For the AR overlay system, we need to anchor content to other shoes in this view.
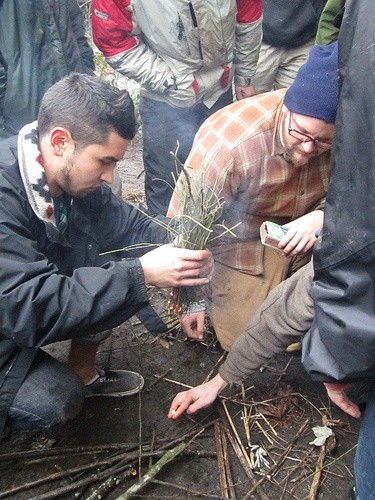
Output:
[285,340,301,352]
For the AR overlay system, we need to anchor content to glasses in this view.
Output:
[287,110,333,150]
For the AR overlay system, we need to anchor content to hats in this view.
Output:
[282,40,339,123]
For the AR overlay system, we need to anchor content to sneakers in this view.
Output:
[82,366,145,396]
[7,428,58,450]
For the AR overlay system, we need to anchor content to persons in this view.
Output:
[297,0,375,500]
[0,72,215,445]
[166,258,317,419]
[250,0,328,94]
[0,0,123,200]
[89,0,264,219]
[165,40,342,352]
